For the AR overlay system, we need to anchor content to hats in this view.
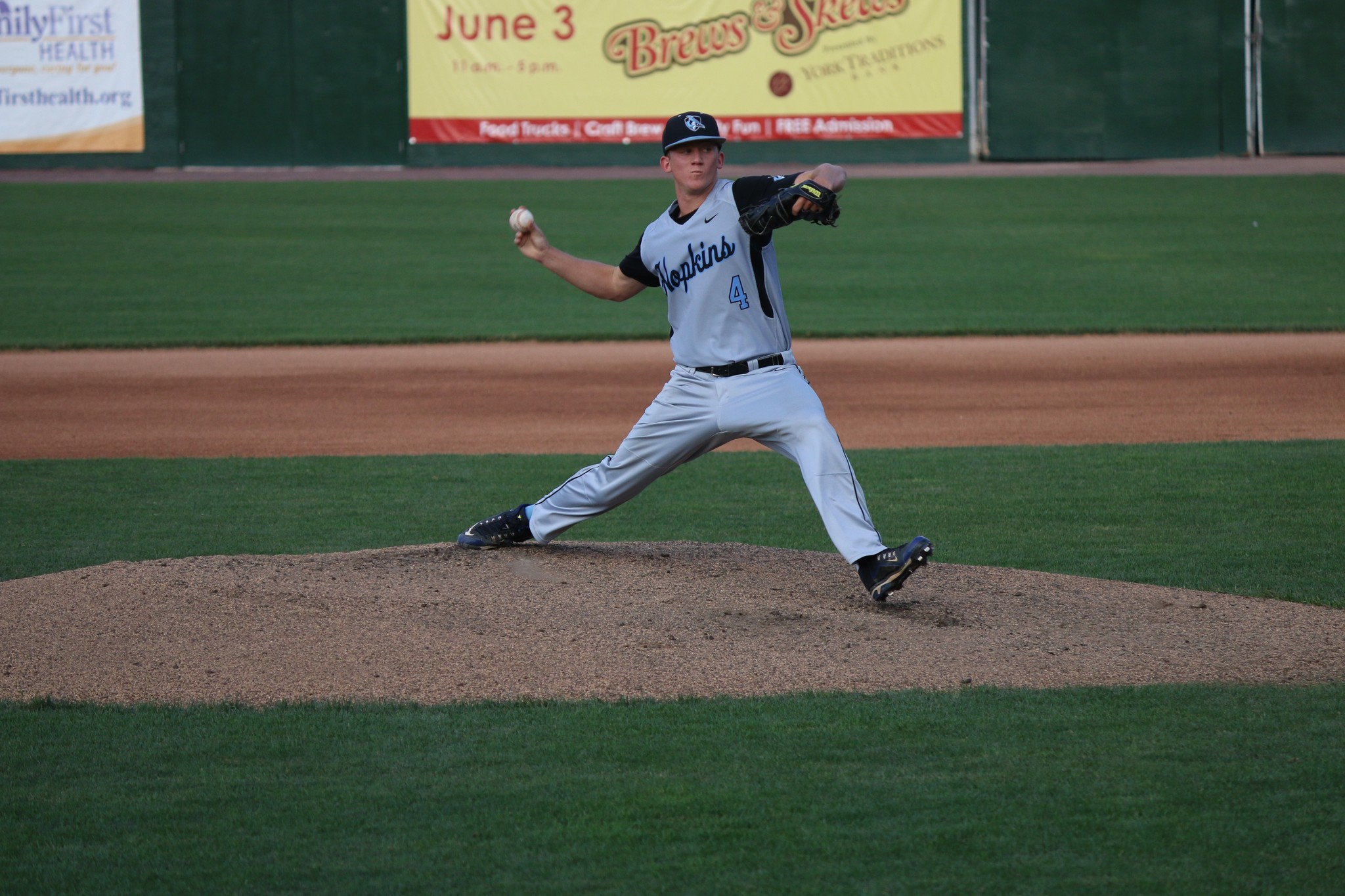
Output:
[662,111,727,155]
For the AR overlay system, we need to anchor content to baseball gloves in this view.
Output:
[738,179,840,244]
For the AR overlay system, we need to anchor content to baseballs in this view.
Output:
[510,207,533,234]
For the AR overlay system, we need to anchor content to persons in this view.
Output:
[456,109,935,602]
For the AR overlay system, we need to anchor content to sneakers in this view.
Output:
[855,536,935,602]
[457,504,534,550]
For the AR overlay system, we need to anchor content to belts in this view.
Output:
[685,354,784,377]
